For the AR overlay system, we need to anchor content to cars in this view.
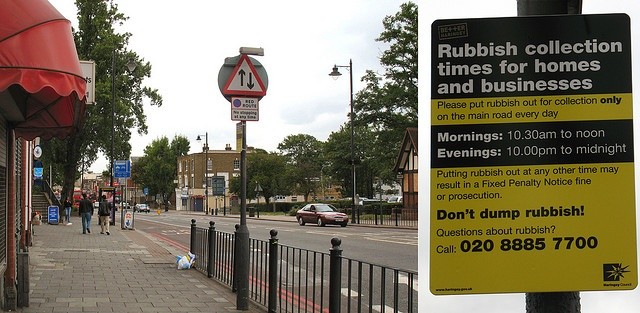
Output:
[134,203,150,213]
[297,204,348,227]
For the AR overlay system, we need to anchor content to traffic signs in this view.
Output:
[231,97,259,121]
[114,160,130,178]
[34,168,43,177]
[48,205,59,223]
[144,188,148,195]
[218,55,268,102]
[223,54,266,96]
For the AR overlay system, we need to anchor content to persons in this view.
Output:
[97,195,112,236]
[78,193,94,234]
[64,197,72,223]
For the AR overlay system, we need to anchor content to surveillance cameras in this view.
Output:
[239,47,264,56]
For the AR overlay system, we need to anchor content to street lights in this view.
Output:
[196,132,208,214]
[329,59,356,223]
